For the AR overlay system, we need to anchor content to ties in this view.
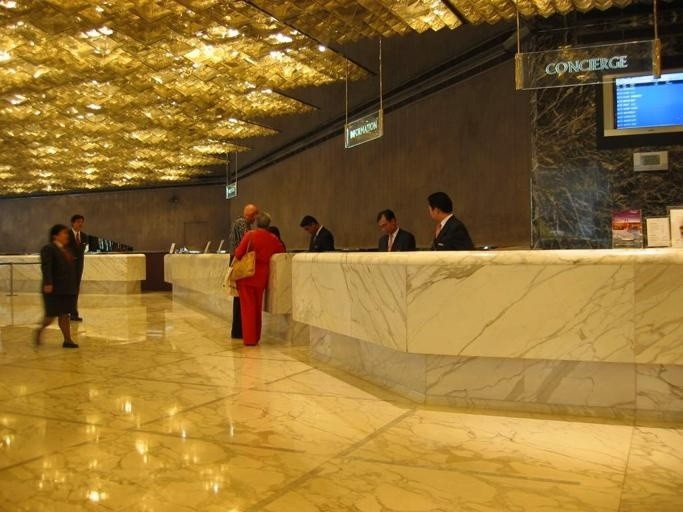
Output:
[388,234,393,251]
[76,232,80,247]
[435,223,441,238]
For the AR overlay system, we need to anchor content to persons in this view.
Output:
[64,214,87,321]
[426,192,475,250]
[228,204,286,346]
[300,215,334,251]
[377,210,415,251]
[31,224,79,348]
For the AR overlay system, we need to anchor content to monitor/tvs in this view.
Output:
[601,67,683,137]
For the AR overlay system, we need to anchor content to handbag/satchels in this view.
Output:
[229,250,257,281]
[222,268,240,297]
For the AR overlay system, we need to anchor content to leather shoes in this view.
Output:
[62,341,79,348]
[70,315,82,322]
